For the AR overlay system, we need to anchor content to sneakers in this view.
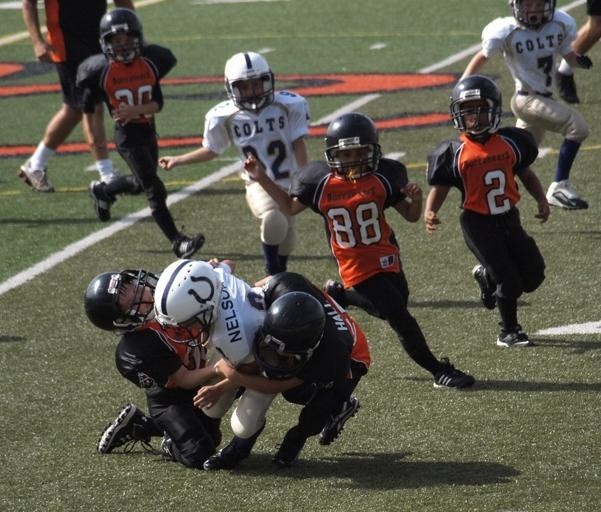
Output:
[171,232,205,260]
[545,178,589,210]
[554,64,581,104]
[15,163,55,193]
[318,395,360,446]
[324,278,346,311]
[89,179,117,223]
[472,263,498,310]
[158,411,222,460]
[275,425,307,466]
[496,327,534,348]
[96,403,150,455]
[432,362,476,390]
[203,419,267,472]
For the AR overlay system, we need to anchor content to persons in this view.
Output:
[551,0,601,103]
[153,255,280,472]
[242,112,477,391]
[76,7,204,261]
[208,273,372,469]
[157,51,310,278]
[85,268,220,470]
[457,0,590,210]
[17,0,137,194]
[423,73,550,349]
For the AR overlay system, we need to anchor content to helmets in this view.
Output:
[252,291,327,381]
[223,50,276,112]
[323,113,383,184]
[84,268,160,334]
[97,7,145,65]
[508,0,558,31]
[449,76,502,142]
[152,258,222,351]
